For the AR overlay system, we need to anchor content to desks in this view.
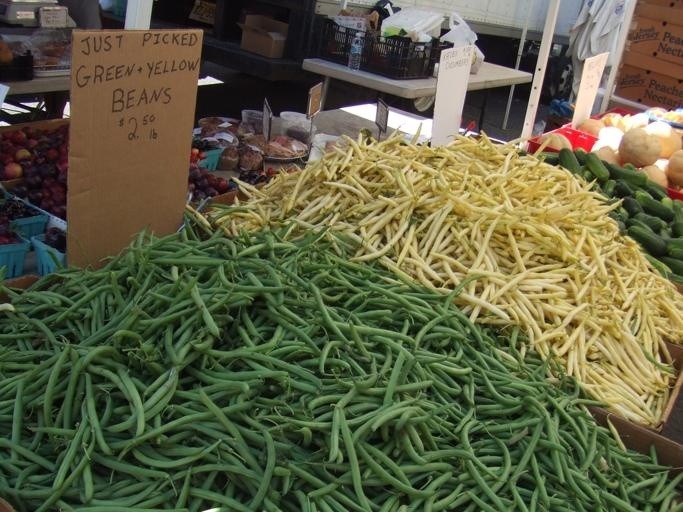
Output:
[306,58,533,135]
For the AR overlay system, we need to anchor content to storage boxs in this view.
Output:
[586,336,683,469]
[612,0,683,118]
[235,12,290,60]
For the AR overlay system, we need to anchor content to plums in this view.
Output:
[0,125,69,220]
[187,147,236,204]
[239,166,276,187]
[191,139,218,152]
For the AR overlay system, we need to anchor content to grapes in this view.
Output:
[0,198,42,226]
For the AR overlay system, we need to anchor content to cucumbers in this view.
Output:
[539,147,683,284]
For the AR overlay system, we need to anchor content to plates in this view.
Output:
[246,137,310,160]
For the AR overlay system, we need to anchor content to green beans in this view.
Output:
[0,196,682,512]
[185,119,682,432]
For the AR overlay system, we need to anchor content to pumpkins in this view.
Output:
[537,133,573,152]
[576,106,683,136]
[596,121,683,188]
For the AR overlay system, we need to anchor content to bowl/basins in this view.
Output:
[280,112,308,121]
[242,110,267,133]
[284,122,317,141]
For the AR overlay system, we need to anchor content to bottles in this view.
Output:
[346,32,363,72]
